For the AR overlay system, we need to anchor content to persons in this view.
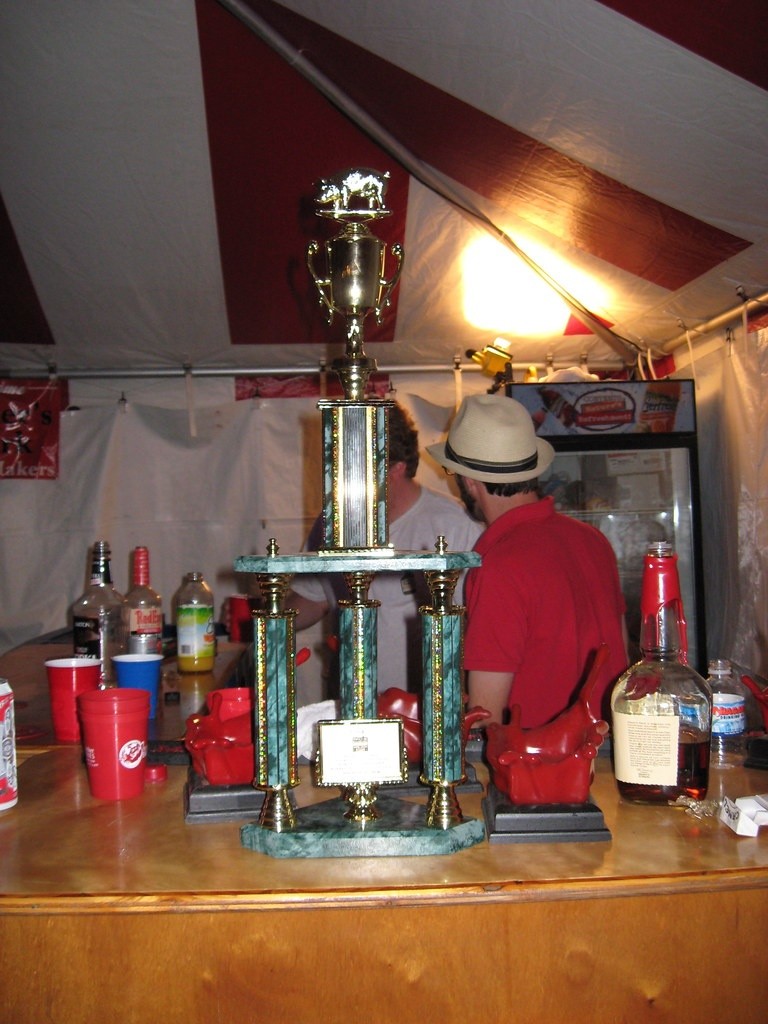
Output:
[463,396,630,729]
[224,395,484,693]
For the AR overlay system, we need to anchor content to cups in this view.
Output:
[45,658,102,742]
[111,654,165,720]
[207,687,255,718]
[79,688,151,800]
[230,597,261,642]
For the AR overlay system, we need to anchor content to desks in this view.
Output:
[0,619,767,1024]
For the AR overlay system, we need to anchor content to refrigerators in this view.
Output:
[505,379,706,686]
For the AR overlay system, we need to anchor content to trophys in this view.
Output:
[307,167,405,401]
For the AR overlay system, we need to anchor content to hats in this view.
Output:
[424,395,556,483]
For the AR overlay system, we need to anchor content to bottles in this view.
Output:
[531,407,547,432]
[611,540,713,806]
[546,354,554,375]
[537,386,580,429]
[636,382,681,433]
[120,546,162,654]
[708,659,747,768]
[176,572,216,674]
[72,542,130,687]
[580,351,588,374]
